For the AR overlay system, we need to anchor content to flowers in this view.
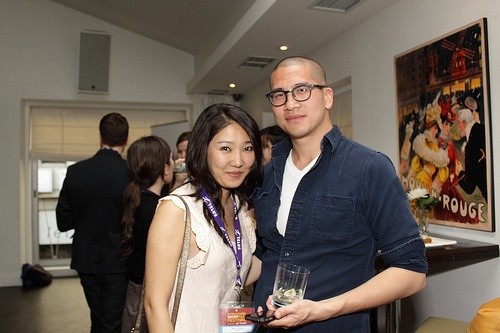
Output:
[407,187,429,211]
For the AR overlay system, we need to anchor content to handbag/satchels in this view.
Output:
[21,264,52,289]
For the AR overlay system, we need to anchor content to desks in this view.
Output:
[371,233,500,333]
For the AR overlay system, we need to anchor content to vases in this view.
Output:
[417,210,430,240]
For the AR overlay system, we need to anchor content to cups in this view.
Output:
[272,262,311,309]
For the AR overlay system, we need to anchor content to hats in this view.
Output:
[465,97,477,110]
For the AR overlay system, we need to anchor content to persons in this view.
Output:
[56,112,134,333]
[230,57,429,333]
[160,125,293,198]
[143,103,257,333]
[121,135,174,285]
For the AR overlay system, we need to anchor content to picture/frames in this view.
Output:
[393,17,496,233]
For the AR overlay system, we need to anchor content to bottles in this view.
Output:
[171,161,188,174]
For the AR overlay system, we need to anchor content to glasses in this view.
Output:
[266,85,329,107]
